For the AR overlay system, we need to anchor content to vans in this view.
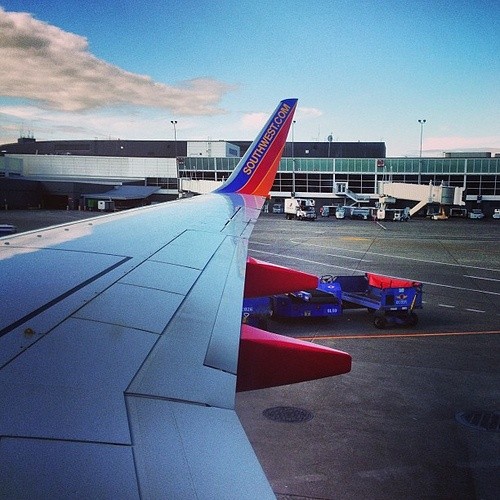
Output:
[273,203,282,214]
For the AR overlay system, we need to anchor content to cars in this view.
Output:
[321,203,499,224]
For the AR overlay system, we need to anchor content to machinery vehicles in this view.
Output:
[240,250,425,331]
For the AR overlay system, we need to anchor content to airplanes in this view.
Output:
[0,96,298,500]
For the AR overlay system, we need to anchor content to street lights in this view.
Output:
[170,119,178,177]
[418,119,426,183]
[289,120,296,194]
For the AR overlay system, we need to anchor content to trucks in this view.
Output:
[284,197,317,222]
